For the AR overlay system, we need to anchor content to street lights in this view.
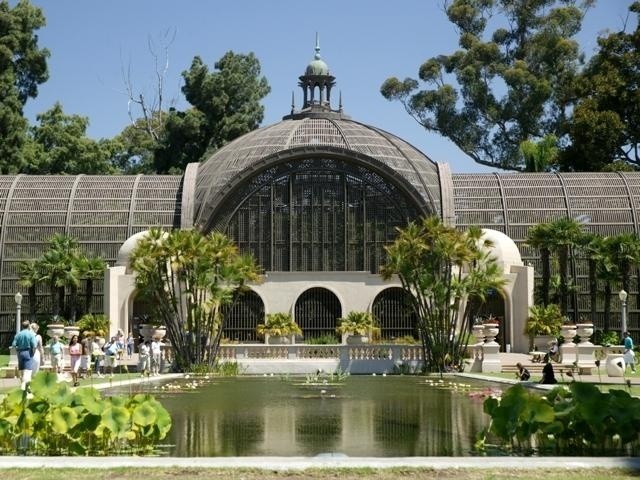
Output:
[15,292,22,332]
[619,290,627,338]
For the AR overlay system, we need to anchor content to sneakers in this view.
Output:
[69,368,162,387]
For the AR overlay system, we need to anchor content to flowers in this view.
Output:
[471,315,499,325]
[523,304,561,353]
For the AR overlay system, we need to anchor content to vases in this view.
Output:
[470,323,499,343]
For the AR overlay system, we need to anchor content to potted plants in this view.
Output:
[333,311,382,344]
[48,312,110,353]
[558,318,594,343]
[138,319,167,342]
[254,311,304,344]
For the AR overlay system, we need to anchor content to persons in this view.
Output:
[12,319,38,399]
[162,334,171,363]
[30,323,44,382]
[138,340,151,377]
[515,362,530,381]
[541,341,558,363]
[151,336,162,377]
[623,332,636,373]
[48,329,135,387]
